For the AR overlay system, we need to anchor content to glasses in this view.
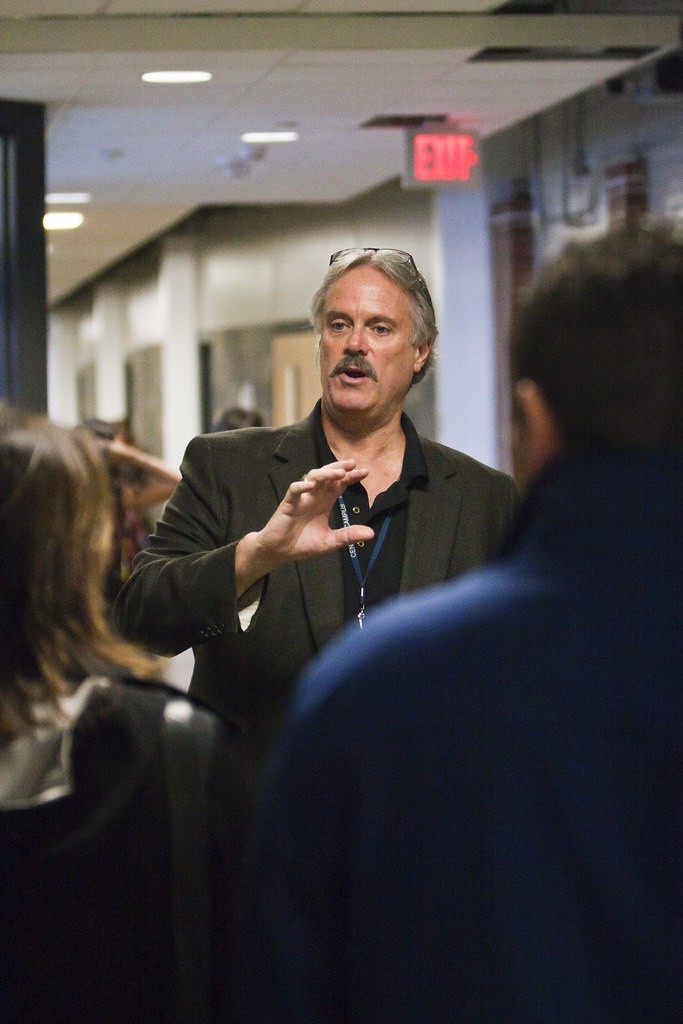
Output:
[328,248,421,280]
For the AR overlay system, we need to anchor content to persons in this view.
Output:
[228,210,682,1024]
[0,402,256,1024]
[108,247,524,776]
